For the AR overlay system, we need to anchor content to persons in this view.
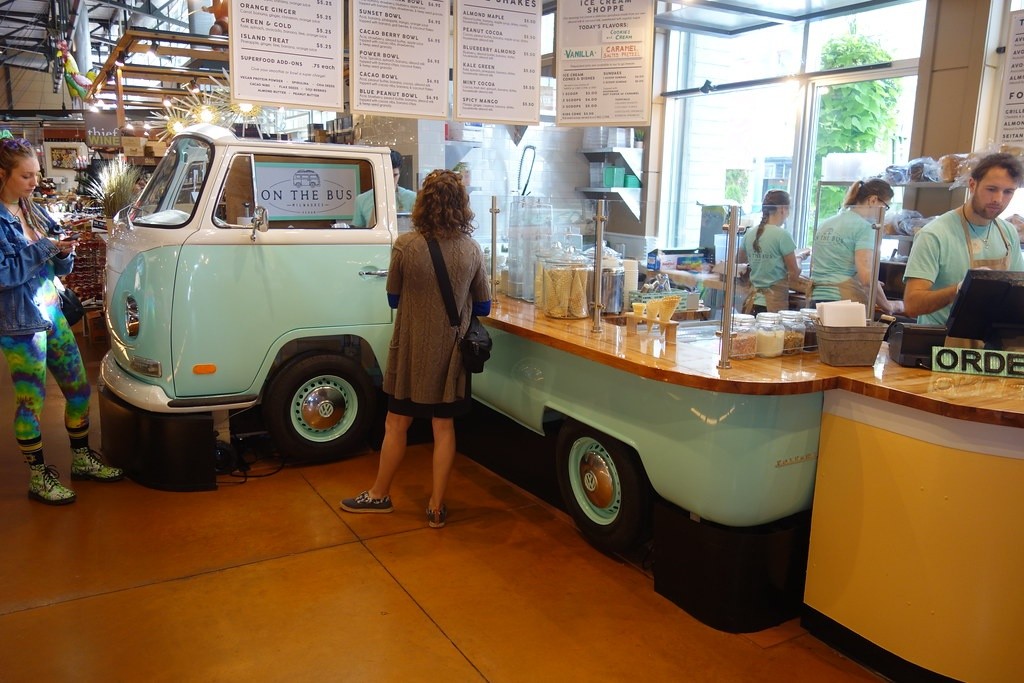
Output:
[0,130,126,506]
[348,150,417,229]
[806,179,905,324]
[903,153,1024,326]
[735,189,810,316]
[339,169,492,528]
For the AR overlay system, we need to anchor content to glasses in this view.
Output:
[868,194,890,212]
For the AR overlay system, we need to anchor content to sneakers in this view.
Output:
[70,445,124,483]
[27,465,77,505]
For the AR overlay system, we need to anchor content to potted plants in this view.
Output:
[79,151,148,239]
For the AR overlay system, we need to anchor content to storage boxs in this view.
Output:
[713,235,740,266]
[647,246,705,277]
[806,313,897,366]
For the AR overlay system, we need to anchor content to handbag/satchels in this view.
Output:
[459,315,493,374]
[58,288,85,326]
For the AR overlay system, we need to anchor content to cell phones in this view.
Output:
[63,233,81,242]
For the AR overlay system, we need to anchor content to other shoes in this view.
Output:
[426,504,447,527]
[340,491,394,513]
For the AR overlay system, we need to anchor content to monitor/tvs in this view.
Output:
[946,268,1024,353]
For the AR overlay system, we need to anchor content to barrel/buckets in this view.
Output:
[714,235,740,264]
[808,313,895,367]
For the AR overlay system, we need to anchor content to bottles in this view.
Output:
[728,308,818,360]
[534,241,624,319]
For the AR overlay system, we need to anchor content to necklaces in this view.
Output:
[0,194,24,223]
[963,204,992,249]
[0,198,19,204]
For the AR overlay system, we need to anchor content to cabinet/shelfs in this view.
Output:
[814,180,971,303]
